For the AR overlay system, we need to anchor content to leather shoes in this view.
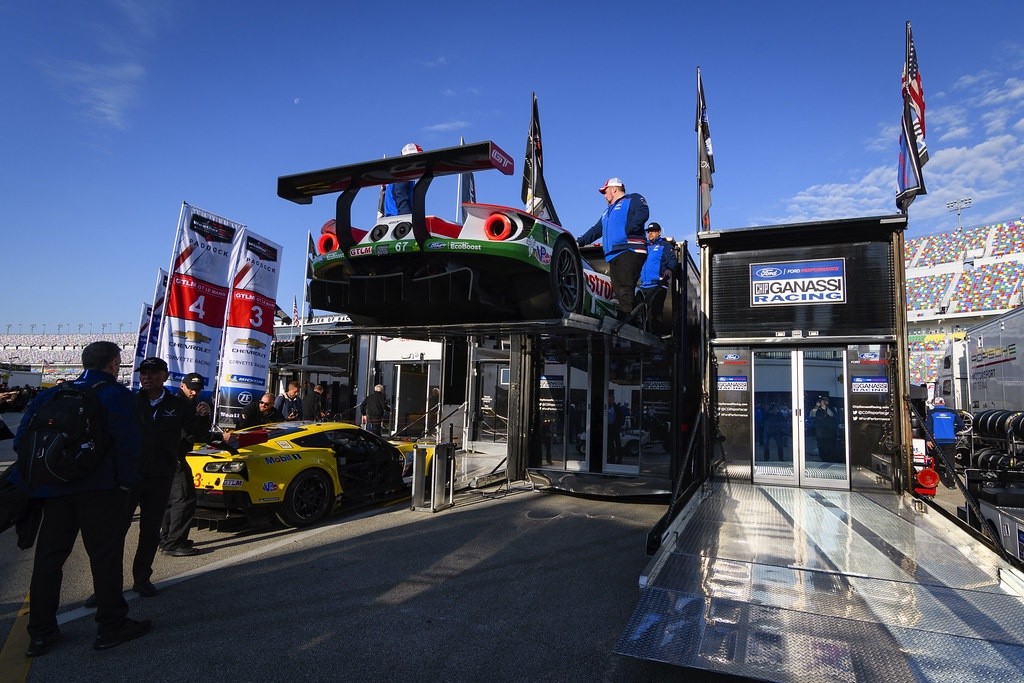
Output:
[159,540,193,551]
[94,619,153,648]
[133,580,158,596]
[163,545,199,555]
[85,592,97,608]
[26,625,60,654]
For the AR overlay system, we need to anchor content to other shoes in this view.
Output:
[949,485,958,490]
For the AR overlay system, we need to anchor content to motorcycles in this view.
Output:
[575,416,655,458]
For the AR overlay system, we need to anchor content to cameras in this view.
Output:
[818,395,822,402]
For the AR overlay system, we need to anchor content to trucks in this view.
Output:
[931,306,1024,443]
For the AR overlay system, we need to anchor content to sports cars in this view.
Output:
[184,420,459,535]
[276,141,624,321]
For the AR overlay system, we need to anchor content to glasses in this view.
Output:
[259,400,271,405]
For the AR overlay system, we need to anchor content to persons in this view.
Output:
[302,385,326,420]
[359,384,391,436]
[11,340,151,656]
[925,397,964,491]
[382,142,424,215]
[569,390,630,464]
[754,402,791,461]
[85,357,211,608]
[55,378,65,385]
[575,177,650,324]
[636,223,677,334]
[275,381,304,420]
[158,372,239,556]
[811,396,838,461]
[237,392,284,431]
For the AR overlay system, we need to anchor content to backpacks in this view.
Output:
[20,380,115,485]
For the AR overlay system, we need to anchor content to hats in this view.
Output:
[182,371,204,389]
[645,223,661,230]
[934,397,945,405]
[134,356,167,372]
[599,177,624,194]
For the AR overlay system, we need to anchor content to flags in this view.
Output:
[895,25,930,214]
[304,234,319,321]
[461,171,476,223]
[293,300,299,328]
[694,76,715,240]
[43,360,49,368]
[521,97,559,226]
